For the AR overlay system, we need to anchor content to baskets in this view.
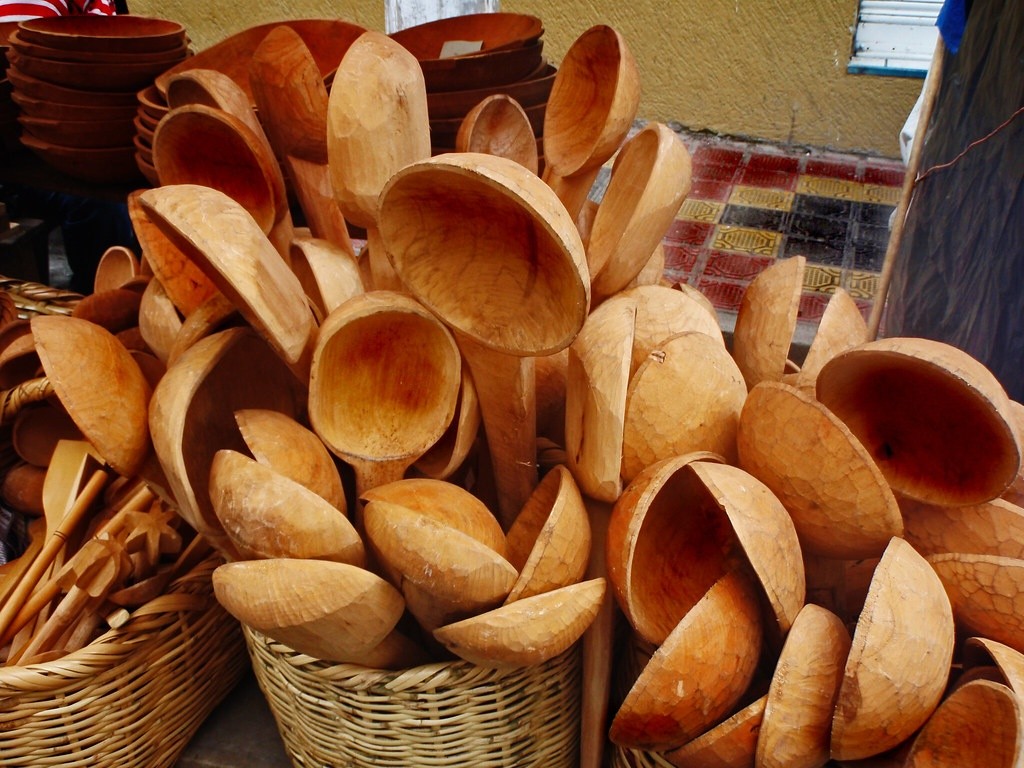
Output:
[239,618,582,768]
[607,622,671,767]
[0,549,246,768]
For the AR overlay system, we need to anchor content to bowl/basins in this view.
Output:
[0,13,563,242]
